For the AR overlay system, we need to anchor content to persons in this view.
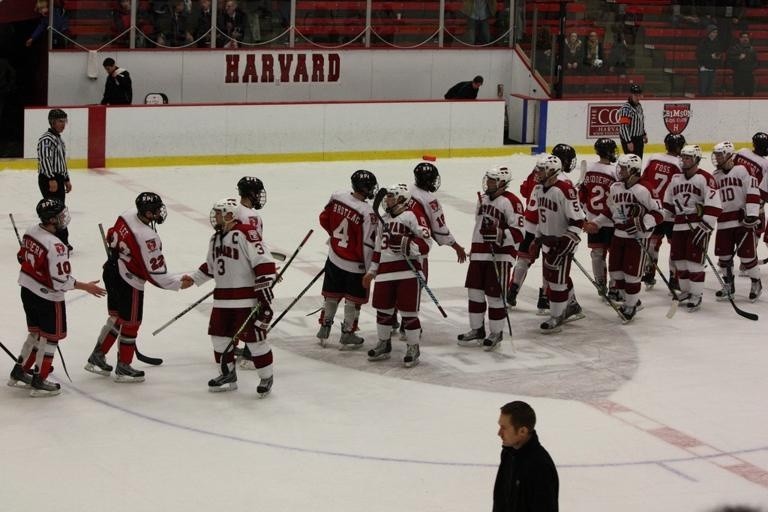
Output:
[179,176,284,398]
[84,193,189,382]
[463,1,758,101]
[116,0,289,52]
[8,199,108,397]
[99,57,133,106]
[37,109,74,252]
[445,76,483,100]
[458,84,768,352]
[493,401,558,512]
[26,2,73,50]
[317,162,465,366]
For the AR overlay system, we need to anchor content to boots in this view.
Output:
[10,355,33,384]
[538,288,583,329]
[87,342,113,371]
[257,375,273,392]
[458,282,520,350]
[749,277,762,299]
[32,365,61,391]
[317,320,422,364]
[115,352,145,377]
[208,358,237,386]
[716,276,735,297]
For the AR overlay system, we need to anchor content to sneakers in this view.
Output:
[669,271,702,308]
[598,274,656,320]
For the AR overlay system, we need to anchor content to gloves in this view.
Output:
[743,218,762,232]
[252,279,274,331]
[388,235,410,256]
[479,218,499,243]
[624,203,647,236]
[690,222,713,249]
[558,231,581,256]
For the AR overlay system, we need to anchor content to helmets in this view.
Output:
[534,144,577,185]
[482,165,511,193]
[237,176,267,209]
[48,109,68,127]
[594,138,645,190]
[665,132,768,172]
[36,198,71,231]
[209,199,239,235]
[351,162,442,214]
[135,192,167,224]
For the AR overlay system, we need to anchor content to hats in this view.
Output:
[631,84,642,93]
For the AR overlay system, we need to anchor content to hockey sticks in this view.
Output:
[372,188,448,320]
[637,239,684,319]
[219,230,312,374]
[98,223,163,366]
[675,199,757,321]
[9,214,74,384]
[152,251,287,339]
[477,190,515,349]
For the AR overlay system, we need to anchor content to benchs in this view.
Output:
[514,1,767,100]
[62,2,509,47]
